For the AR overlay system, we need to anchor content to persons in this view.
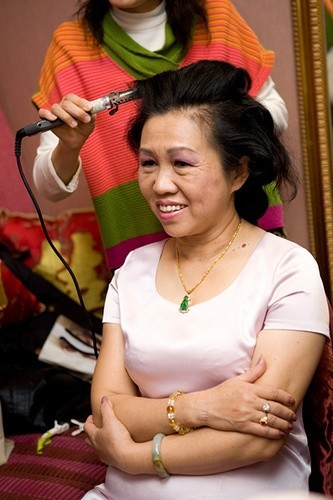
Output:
[76,56,333,500]
[27,0,297,293]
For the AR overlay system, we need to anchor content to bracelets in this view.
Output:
[151,431,172,480]
[164,390,194,435]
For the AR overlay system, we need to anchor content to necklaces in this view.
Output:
[174,217,244,314]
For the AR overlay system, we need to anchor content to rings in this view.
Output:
[259,412,270,426]
[262,400,271,413]
